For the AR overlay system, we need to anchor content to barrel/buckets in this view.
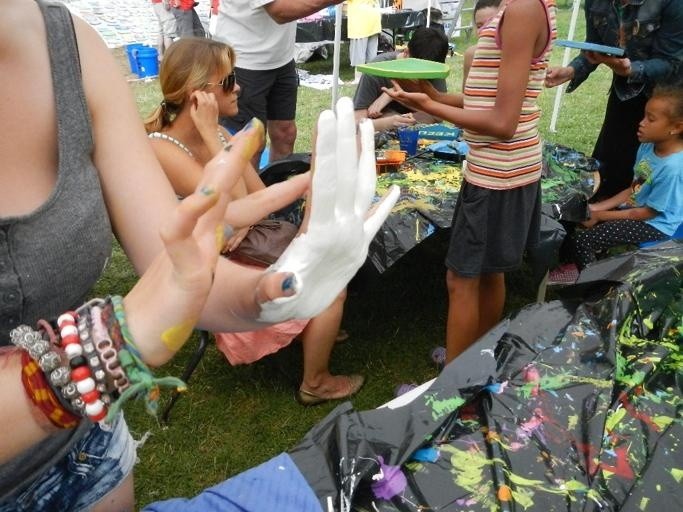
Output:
[135,47,158,79]
[127,43,142,72]
[257,146,270,170]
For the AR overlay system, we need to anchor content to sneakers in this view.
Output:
[429,347,447,364]
[394,383,418,397]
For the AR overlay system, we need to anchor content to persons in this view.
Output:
[145,2,365,407]
[382,0,556,399]
[1,0,401,512]
[347,1,450,129]
[542,1,683,289]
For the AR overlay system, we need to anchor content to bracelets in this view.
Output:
[12,294,157,436]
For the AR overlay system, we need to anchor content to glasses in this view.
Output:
[205,70,237,95]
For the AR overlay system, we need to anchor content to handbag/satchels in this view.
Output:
[229,218,299,267]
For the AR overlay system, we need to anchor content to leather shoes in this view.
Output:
[296,374,364,408]
